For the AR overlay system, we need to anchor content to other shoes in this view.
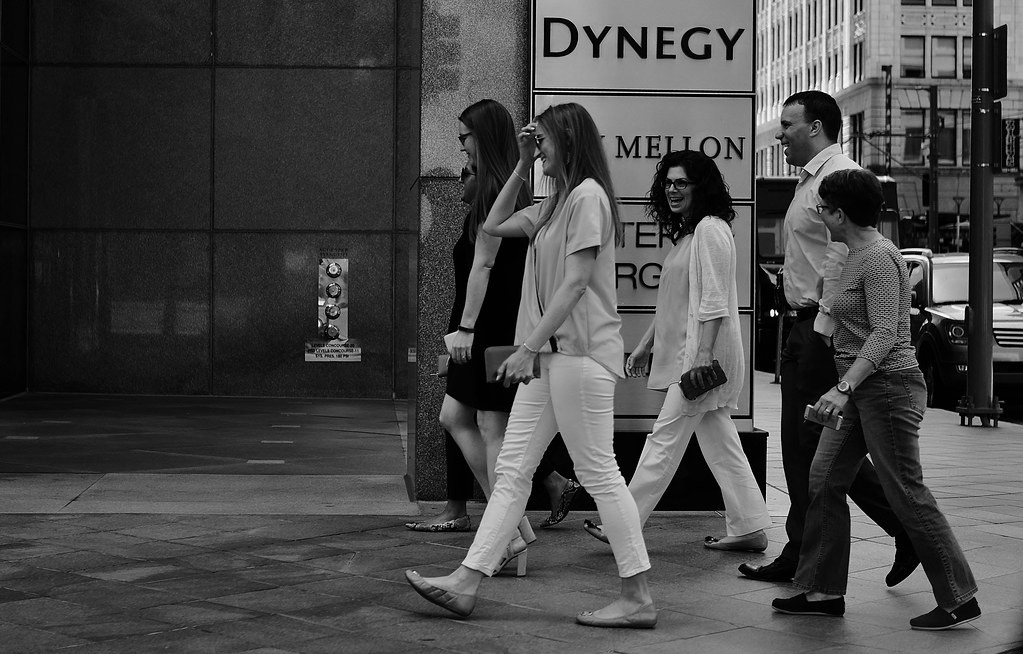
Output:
[405,516,471,533]
[540,478,586,527]
[583,520,611,545]
[885,529,921,586]
[403,571,475,617]
[739,564,794,581]
[704,531,768,551]
[577,603,658,628]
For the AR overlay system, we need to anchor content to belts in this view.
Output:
[789,304,821,321]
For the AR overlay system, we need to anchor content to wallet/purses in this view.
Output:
[438,355,451,376]
[680,361,727,400]
[483,346,541,383]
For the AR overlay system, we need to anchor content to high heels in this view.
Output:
[491,534,527,577]
[518,518,536,545]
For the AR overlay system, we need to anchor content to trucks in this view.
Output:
[754,171,905,371]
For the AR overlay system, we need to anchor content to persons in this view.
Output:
[584,150,773,552]
[412,99,578,576]
[771,168,982,630]
[740,89,919,587]
[406,104,658,629]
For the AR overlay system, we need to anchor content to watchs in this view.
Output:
[835,380,853,396]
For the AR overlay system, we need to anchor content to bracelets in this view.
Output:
[513,171,526,183]
[456,326,477,335]
[523,342,540,354]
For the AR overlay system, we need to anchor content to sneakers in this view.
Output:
[769,592,845,616]
[910,598,983,631]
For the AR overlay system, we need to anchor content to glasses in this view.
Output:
[457,132,475,146]
[660,178,692,189]
[460,168,477,180]
[535,135,548,145]
[817,204,832,213]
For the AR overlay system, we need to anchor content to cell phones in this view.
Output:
[804,404,843,431]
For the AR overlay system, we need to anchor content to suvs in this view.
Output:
[895,246,1023,410]
